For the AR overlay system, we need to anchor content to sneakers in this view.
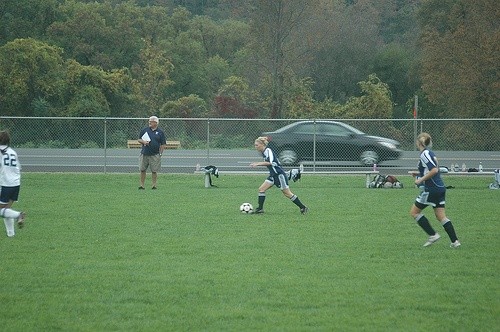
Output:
[300,207,308,215]
[251,208,264,214]
[450,239,461,248]
[424,233,441,247]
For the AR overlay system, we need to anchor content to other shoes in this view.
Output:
[152,186,157,189]
[17,212,26,228]
[138,186,145,190]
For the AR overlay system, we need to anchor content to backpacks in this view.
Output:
[369,173,386,188]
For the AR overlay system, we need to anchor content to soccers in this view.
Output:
[239,203,254,215]
[369,174,404,189]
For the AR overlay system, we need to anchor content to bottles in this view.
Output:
[451,162,459,172]
[300,164,303,172]
[462,163,467,171]
[479,162,483,172]
[373,164,376,172]
[196,163,200,170]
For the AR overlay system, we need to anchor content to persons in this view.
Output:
[408,133,461,248]
[0,131,25,237]
[250,137,308,215]
[139,116,166,190]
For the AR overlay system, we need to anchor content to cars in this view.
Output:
[259,120,403,168]
